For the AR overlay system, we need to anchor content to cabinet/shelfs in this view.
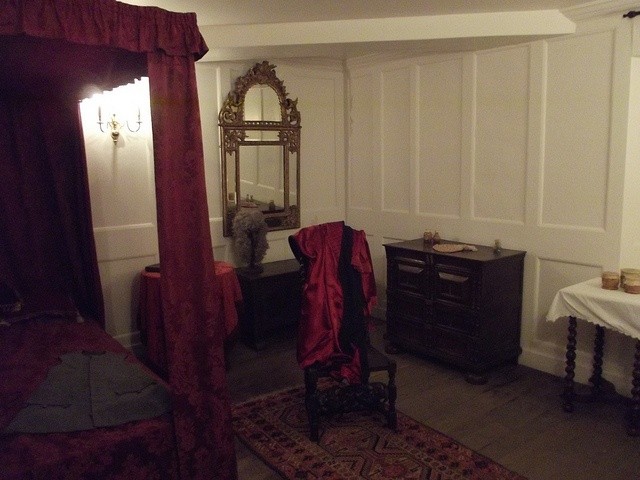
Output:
[381,236,526,385]
[233,258,299,346]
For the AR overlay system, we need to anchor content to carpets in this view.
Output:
[233,375,530,479]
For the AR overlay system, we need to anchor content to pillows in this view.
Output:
[0,277,80,326]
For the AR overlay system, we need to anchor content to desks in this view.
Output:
[546,276,639,438]
[142,260,237,369]
[240,198,284,214]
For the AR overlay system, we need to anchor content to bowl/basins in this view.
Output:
[623,276,640,294]
[602,272,619,290]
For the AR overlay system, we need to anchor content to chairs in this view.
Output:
[298,224,397,442]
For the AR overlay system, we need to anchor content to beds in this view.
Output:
[2,0,234,478]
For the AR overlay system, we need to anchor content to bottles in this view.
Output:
[620,268,639,289]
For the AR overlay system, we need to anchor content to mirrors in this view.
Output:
[218,60,303,237]
